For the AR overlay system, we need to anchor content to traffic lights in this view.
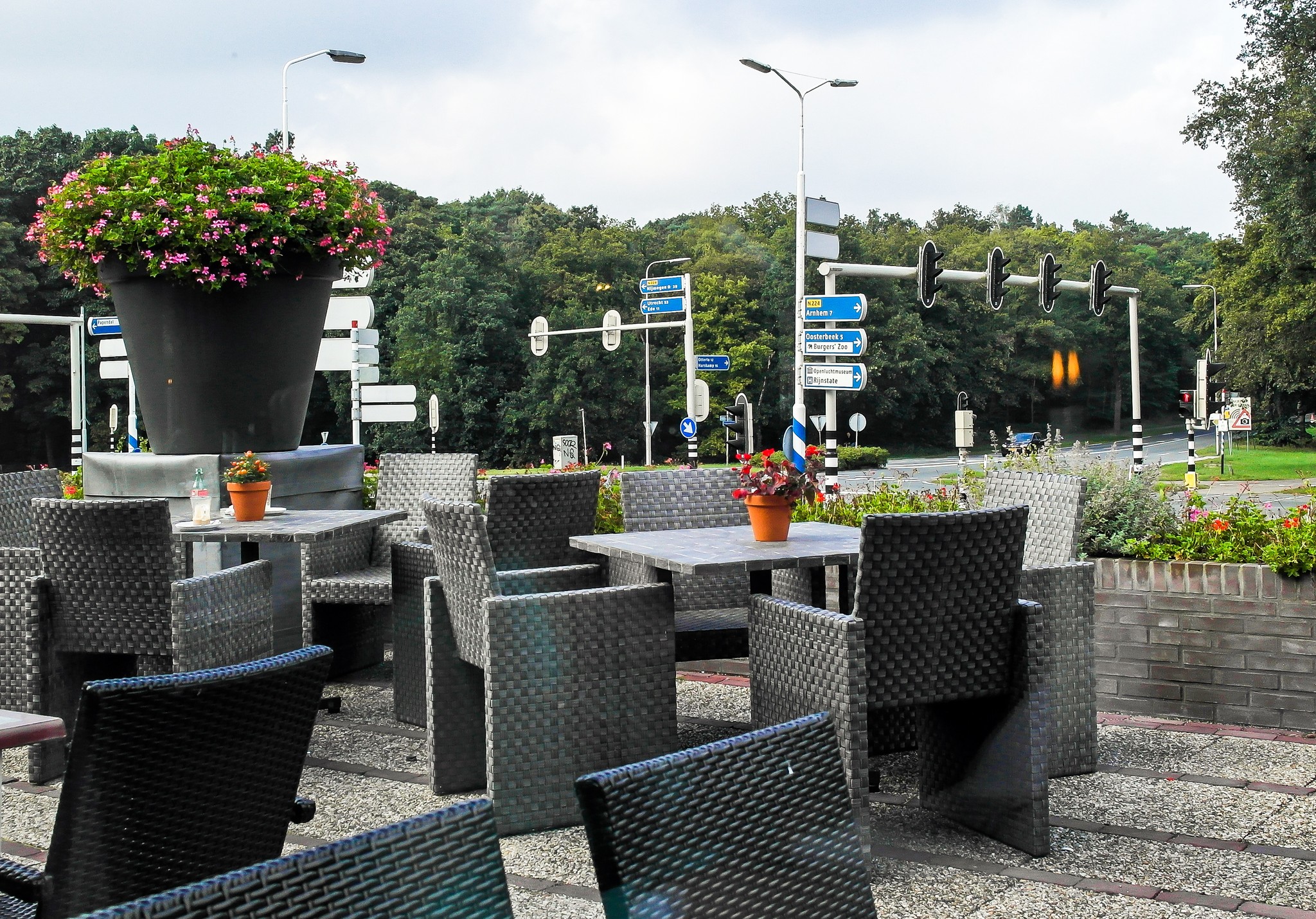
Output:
[1205,348,1226,431]
[1041,253,1062,313]
[1092,260,1113,317]
[1180,390,1196,419]
[921,240,943,309]
[989,246,1012,311]
[723,393,749,453]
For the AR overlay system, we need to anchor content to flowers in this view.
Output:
[218,451,272,484]
[25,123,392,299]
[732,444,828,510]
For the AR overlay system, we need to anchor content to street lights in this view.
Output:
[282,49,366,154]
[738,56,858,471]
[645,257,693,465]
[1183,285,1221,455]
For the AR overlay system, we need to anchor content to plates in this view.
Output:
[264,508,286,513]
[175,521,221,531]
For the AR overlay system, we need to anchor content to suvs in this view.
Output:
[1002,432,1043,457]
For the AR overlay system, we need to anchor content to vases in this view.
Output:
[743,495,797,541]
[97,256,343,453]
[227,481,271,521]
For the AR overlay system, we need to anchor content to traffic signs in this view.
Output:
[639,275,686,315]
[697,355,730,371]
[800,294,867,391]
[87,317,122,335]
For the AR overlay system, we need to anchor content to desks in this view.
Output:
[0,709,67,750]
[568,521,861,599]
[82,446,364,506]
[172,510,408,564]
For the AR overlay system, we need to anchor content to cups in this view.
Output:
[190,497,211,523]
[266,485,272,507]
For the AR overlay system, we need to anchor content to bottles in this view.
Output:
[191,470,208,498]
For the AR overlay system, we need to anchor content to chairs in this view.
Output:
[0,454,1097,919]
